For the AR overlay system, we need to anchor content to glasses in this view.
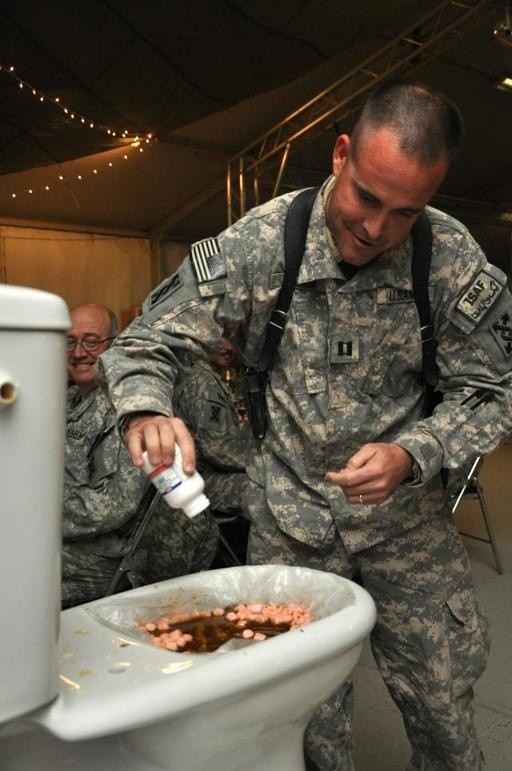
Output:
[66,336,116,352]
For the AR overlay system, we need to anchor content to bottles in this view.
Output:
[134,445,213,519]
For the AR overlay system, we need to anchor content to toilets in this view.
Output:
[1,283,377,771]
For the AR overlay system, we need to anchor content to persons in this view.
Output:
[100,82,511,770]
[62,298,257,608]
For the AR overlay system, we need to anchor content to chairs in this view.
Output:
[210,507,246,567]
[449,452,506,577]
[104,484,165,599]
